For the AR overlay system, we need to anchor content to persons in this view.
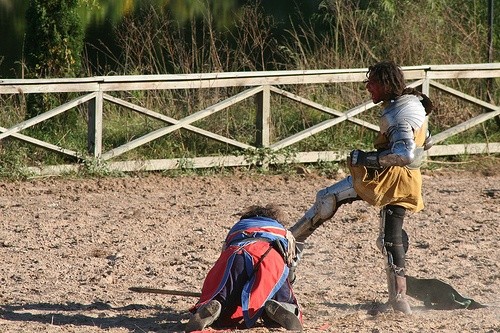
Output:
[289,61,434,316]
[185,203,304,333]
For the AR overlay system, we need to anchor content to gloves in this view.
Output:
[349,147,385,170]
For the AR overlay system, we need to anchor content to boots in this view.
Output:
[368,274,412,316]
[287,214,316,254]
[265,299,304,331]
[183,300,221,333]
